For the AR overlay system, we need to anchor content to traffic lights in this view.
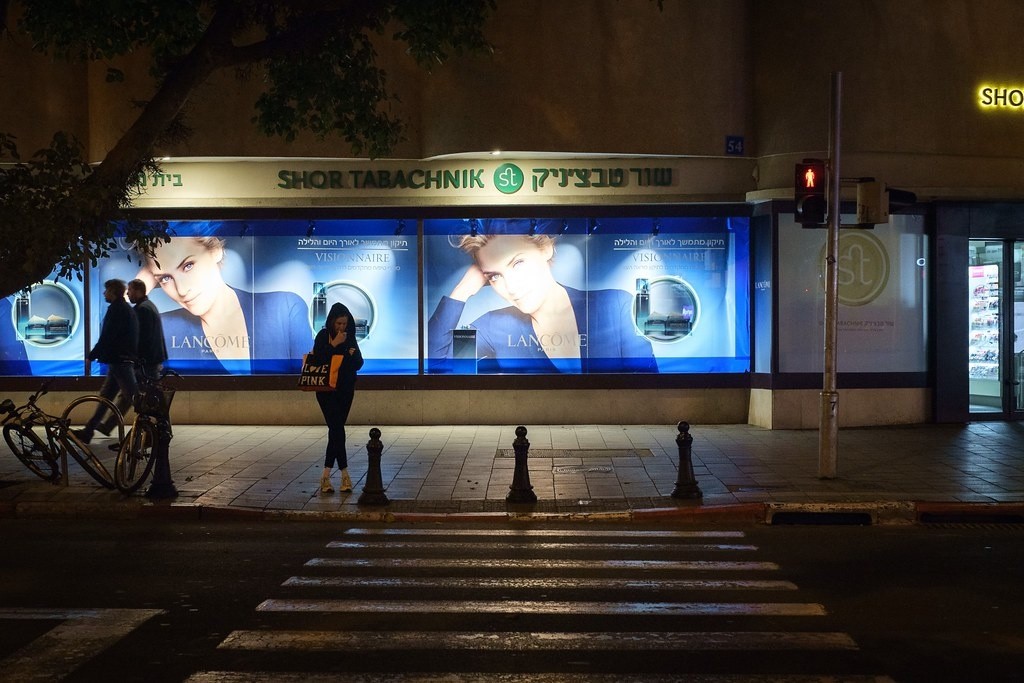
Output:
[793,161,827,224]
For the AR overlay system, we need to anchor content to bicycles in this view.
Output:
[0,376,116,492]
[109,355,186,494]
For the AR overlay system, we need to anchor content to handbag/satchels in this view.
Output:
[299,329,344,391]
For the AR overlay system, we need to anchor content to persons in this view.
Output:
[122,236,313,373]
[70,279,168,452]
[311,302,364,494]
[426,220,655,373]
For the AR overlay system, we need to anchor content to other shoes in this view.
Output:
[96,423,110,436]
[340,475,353,493]
[73,428,91,444]
[320,477,335,492]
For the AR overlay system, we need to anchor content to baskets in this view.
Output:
[133,382,176,418]
